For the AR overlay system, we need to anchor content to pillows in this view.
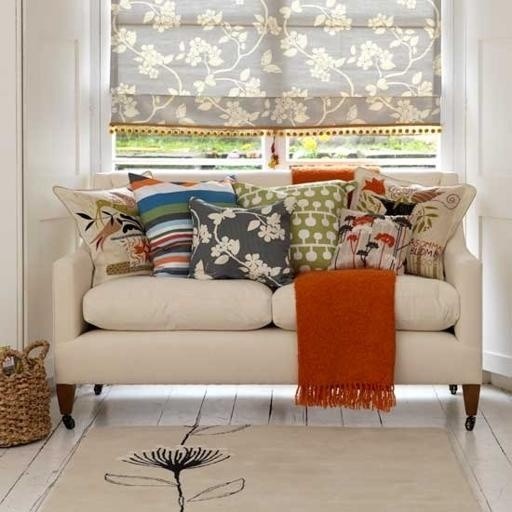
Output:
[351,170,476,280]
[53,171,156,288]
[187,197,295,287]
[331,207,409,275]
[232,179,357,272]
[128,172,238,279]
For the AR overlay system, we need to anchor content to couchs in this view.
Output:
[52,168,484,431]
